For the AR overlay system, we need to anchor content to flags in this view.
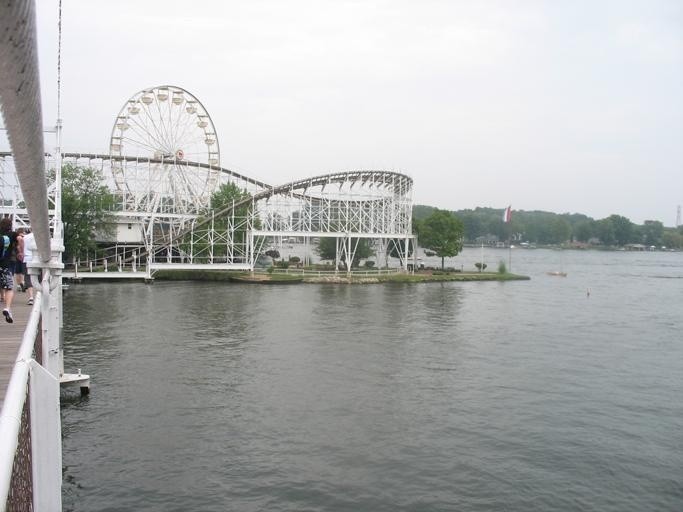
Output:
[502,206,511,224]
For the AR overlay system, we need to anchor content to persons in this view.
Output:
[1,218,34,323]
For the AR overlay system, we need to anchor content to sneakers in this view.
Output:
[2,308,13,323]
[20,283,24,292]
[27,297,33,305]
